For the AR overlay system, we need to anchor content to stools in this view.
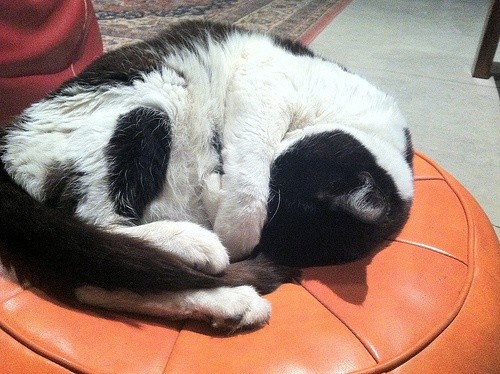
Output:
[0,148,500,374]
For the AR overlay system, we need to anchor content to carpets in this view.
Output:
[88,0,353,53]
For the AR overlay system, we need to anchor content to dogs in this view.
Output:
[0,19,416,337]
